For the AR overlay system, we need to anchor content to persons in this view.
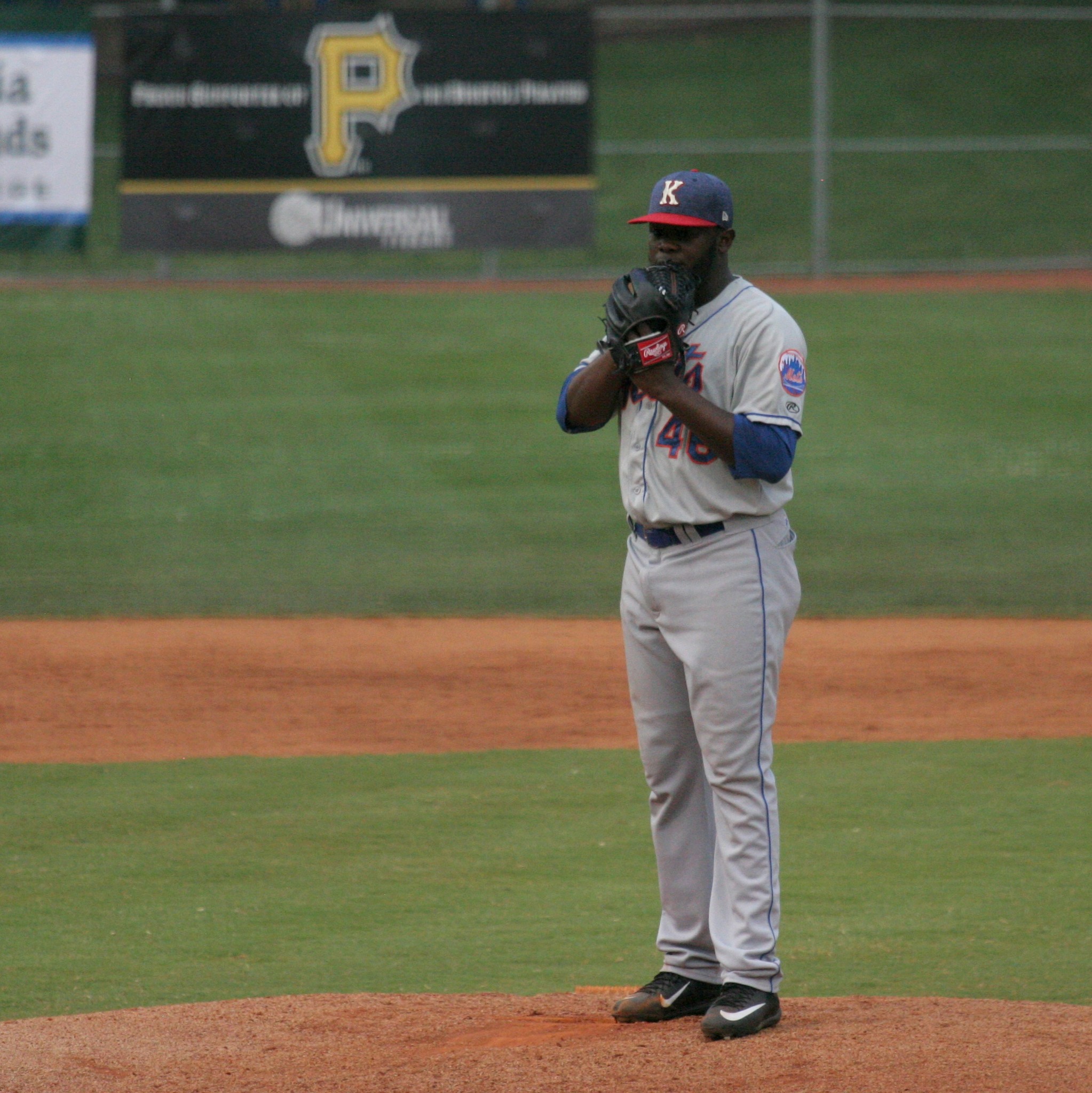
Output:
[556,168,806,1038]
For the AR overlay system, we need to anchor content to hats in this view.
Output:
[628,169,734,231]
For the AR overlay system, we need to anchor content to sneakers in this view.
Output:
[701,983,781,1039]
[610,971,721,1023]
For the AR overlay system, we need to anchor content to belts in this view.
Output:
[628,516,725,550]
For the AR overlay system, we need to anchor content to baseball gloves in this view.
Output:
[598,261,697,381]
